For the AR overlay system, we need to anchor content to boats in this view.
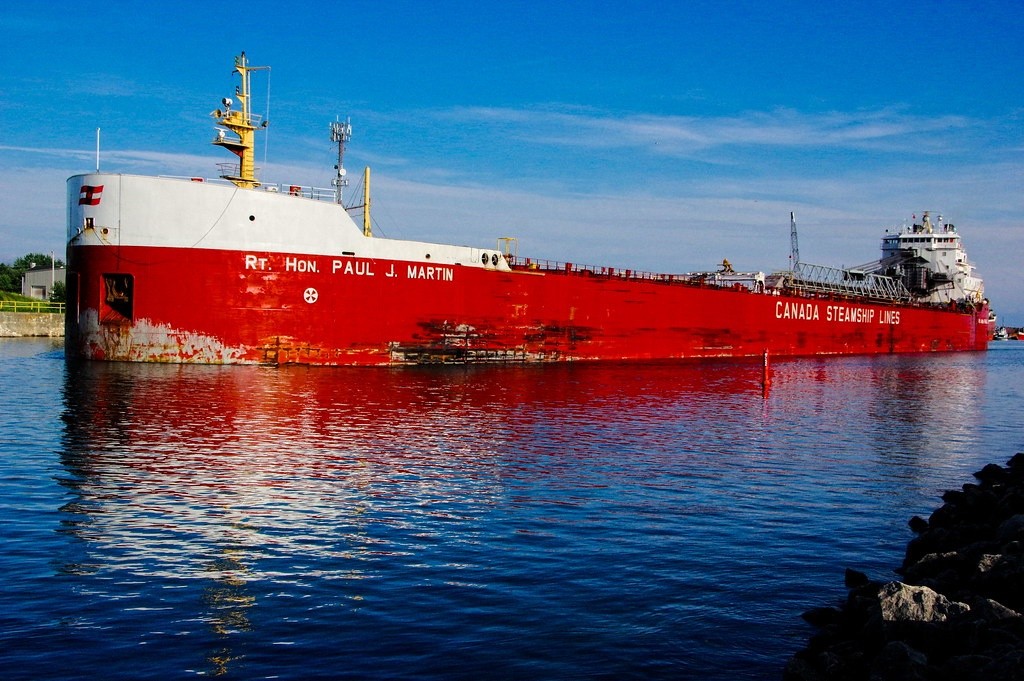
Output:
[994,316,1008,341]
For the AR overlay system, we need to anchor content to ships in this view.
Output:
[62,48,991,369]
